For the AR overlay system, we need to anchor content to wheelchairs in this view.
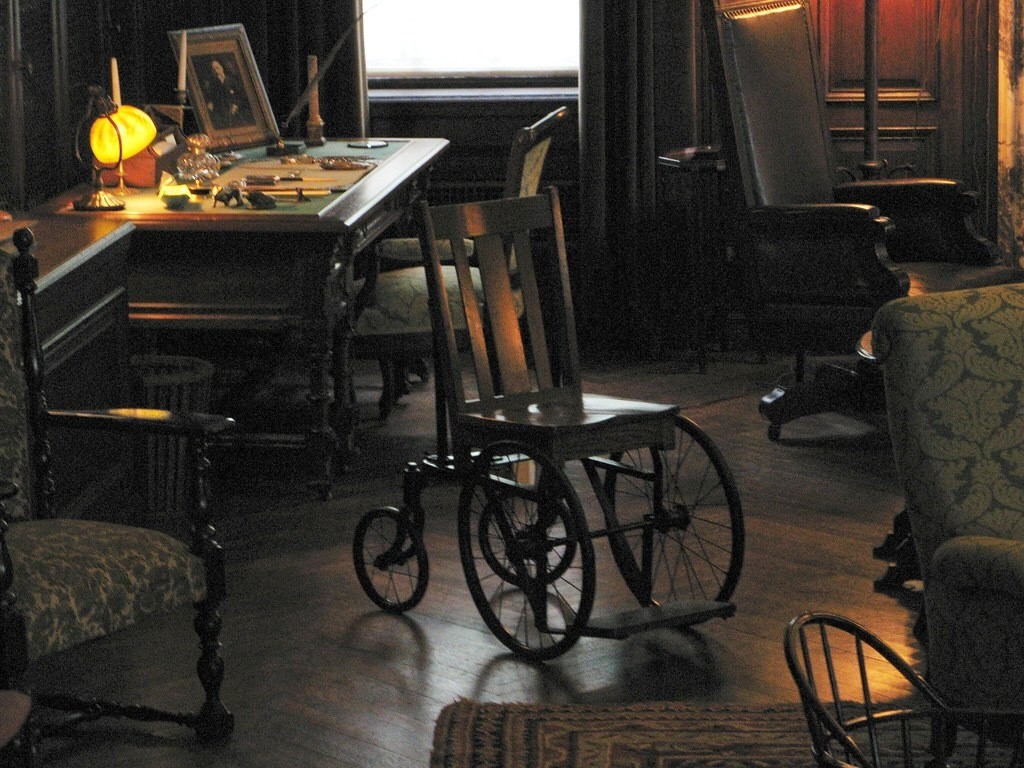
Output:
[353,184,747,662]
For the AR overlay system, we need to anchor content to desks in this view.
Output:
[25,138,451,502]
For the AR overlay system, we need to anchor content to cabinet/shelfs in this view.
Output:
[0,217,137,525]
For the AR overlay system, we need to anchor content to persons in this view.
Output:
[206,58,254,130]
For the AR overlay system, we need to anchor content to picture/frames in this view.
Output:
[167,22,280,154]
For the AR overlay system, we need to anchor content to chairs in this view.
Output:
[327,107,571,474]
[0,229,238,768]
[700,0,1024,442]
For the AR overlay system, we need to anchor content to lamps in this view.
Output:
[73,84,157,210]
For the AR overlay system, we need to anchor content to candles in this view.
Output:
[307,54,323,124]
[176,29,188,89]
[111,57,122,105]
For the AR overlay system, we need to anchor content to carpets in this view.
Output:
[431,697,1024,768]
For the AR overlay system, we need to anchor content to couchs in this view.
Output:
[871,283,1024,745]
